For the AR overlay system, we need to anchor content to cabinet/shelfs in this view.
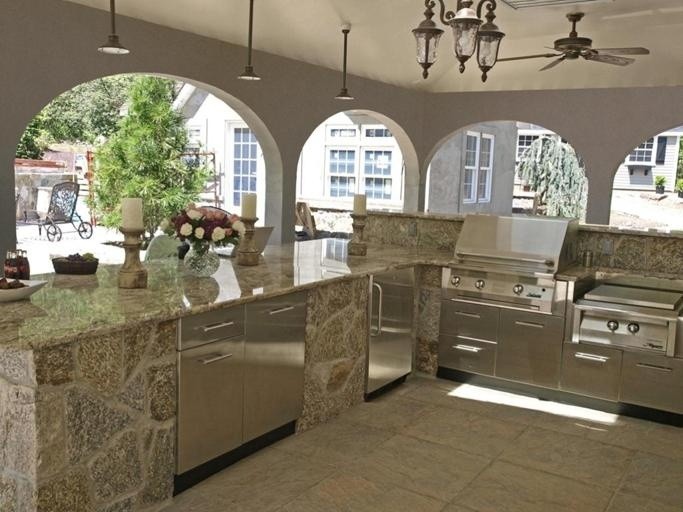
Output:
[365,266,415,395]
[618,348,682,415]
[495,306,564,391]
[177,303,245,477]
[242,289,305,444]
[560,341,624,403]
[438,299,499,379]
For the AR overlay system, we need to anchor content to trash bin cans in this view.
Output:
[35,188,54,219]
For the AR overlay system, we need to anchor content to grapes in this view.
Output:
[67,253,96,262]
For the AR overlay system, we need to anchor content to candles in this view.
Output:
[353,193,366,215]
[120,198,144,229]
[241,191,257,219]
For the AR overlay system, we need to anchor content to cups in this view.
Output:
[4,248,30,278]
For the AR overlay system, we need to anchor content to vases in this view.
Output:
[183,238,220,278]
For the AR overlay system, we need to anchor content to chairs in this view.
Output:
[20,181,93,240]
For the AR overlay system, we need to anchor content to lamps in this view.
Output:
[236,0,261,81]
[96,0,130,55]
[412,0,506,83]
[334,22,355,99]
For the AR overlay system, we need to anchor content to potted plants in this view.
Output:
[655,176,666,194]
[675,178,683,198]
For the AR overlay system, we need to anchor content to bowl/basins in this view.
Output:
[51,257,97,274]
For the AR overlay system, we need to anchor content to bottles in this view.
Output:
[583,250,593,268]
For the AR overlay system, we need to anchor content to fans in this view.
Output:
[495,11,650,72]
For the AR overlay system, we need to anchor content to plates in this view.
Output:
[0,279,48,302]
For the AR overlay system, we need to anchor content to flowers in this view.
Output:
[159,200,247,270]
